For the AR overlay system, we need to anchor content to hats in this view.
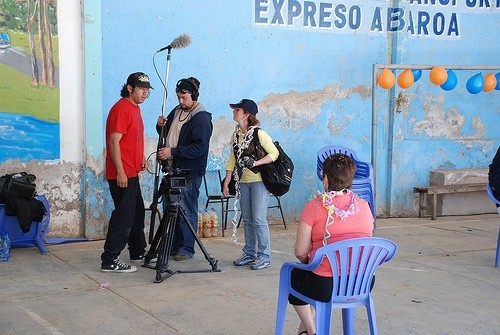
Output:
[127,72,154,89]
[229,99,258,116]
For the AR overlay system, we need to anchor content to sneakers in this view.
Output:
[101,257,138,273]
[130,251,158,263]
[251,259,271,270]
[232,252,254,266]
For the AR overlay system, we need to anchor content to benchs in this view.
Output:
[413,185,486,221]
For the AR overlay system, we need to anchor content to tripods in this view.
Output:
[143,201,219,283]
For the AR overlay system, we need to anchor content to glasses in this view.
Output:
[175,88,191,94]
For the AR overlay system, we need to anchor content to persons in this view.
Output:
[223,99,279,270]
[488,146,500,212]
[288,153,375,335]
[100,71,157,273]
[156,77,213,261]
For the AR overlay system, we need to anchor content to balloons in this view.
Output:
[378,68,395,89]
[482,72,497,92]
[411,64,422,82]
[466,72,484,94]
[494,72,500,90]
[440,70,457,91]
[430,65,447,85]
[397,69,414,89]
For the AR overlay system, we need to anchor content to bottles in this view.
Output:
[197,208,219,238]
[0,232,11,262]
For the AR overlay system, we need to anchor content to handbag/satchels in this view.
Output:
[0,171,38,199]
[253,128,294,197]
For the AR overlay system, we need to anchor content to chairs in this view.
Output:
[204,169,287,237]
[487,183,500,267]
[317,146,375,215]
[276,237,399,335]
[0,195,50,262]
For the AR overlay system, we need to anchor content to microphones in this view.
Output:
[159,31,192,52]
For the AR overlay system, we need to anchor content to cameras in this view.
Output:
[241,156,254,168]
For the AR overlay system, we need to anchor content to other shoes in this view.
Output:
[174,253,191,261]
